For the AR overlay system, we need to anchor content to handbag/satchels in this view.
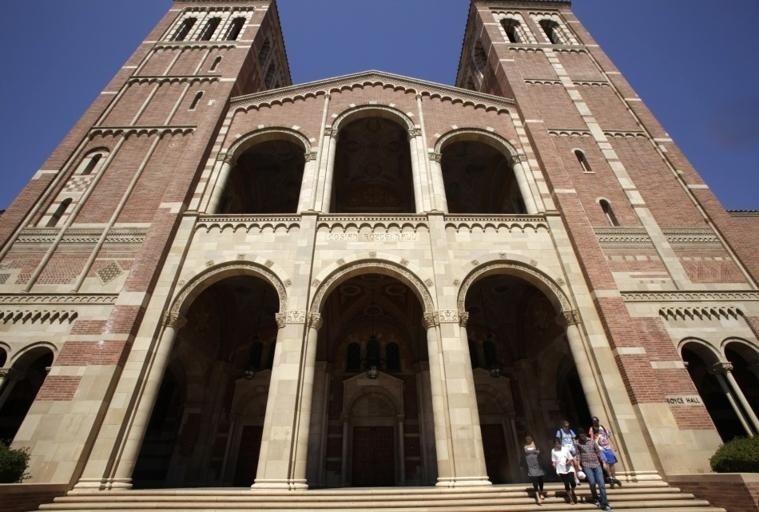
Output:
[577,471,587,479]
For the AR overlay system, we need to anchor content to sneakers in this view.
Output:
[608,477,621,486]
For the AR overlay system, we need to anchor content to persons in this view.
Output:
[588,416,620,485]
[521,433,546,504]
[550,437,578,504]
[574,430,611,511]
[555,421,582,487]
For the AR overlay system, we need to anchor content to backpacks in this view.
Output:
[551,423,571,442]
